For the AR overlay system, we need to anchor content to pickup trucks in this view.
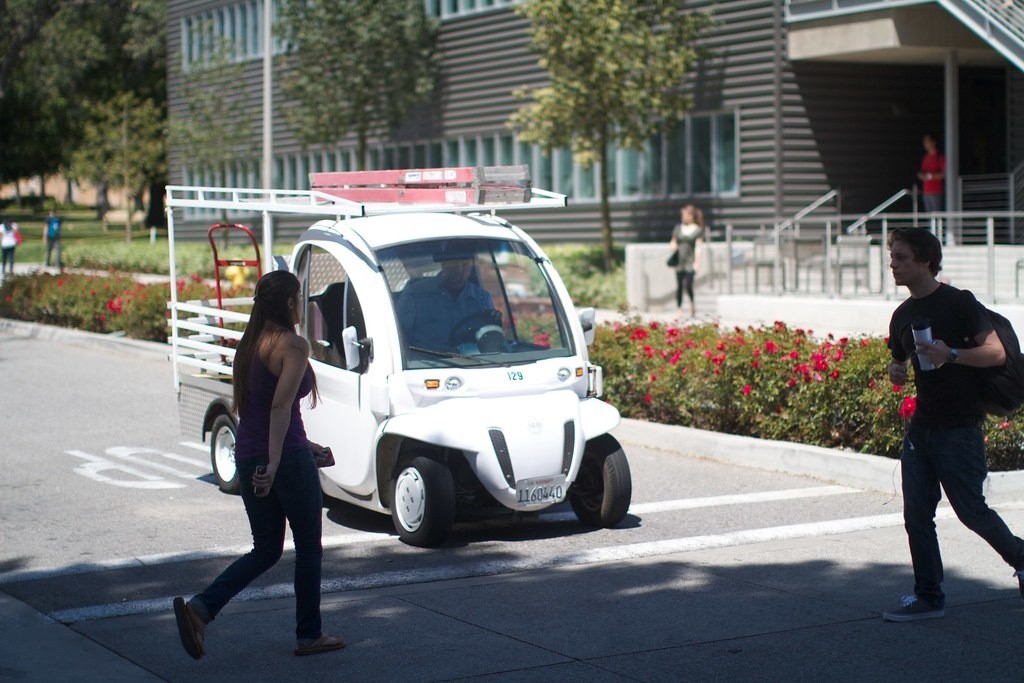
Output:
[165,165,633,544]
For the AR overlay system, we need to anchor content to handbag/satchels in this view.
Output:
[667,250,679,267]
[13,229,23,245]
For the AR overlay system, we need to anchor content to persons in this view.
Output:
[174,270,347,661]
[43,205,63,270]
[672,206,706,322]
[0,217,18,274]
[882,227,1024,623]
[918,133,947,210]
[395,251,511,368]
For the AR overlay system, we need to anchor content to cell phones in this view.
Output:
[254,466,266,494]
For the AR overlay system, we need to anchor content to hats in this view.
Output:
[432,240,477,262]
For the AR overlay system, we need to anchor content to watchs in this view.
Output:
[947,348,959,364]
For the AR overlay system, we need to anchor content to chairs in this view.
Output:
[307,276,436,369]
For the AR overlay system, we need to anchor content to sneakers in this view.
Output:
[883,594,945,622]
[1013,570,1024,600]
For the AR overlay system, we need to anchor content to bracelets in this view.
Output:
[928,172,934,181]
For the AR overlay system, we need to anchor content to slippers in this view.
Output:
[295,632,344,655]
[173,597,206,660]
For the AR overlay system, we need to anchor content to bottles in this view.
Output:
[910,316,935,371]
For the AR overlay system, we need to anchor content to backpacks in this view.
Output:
[951,290,1024,417]
